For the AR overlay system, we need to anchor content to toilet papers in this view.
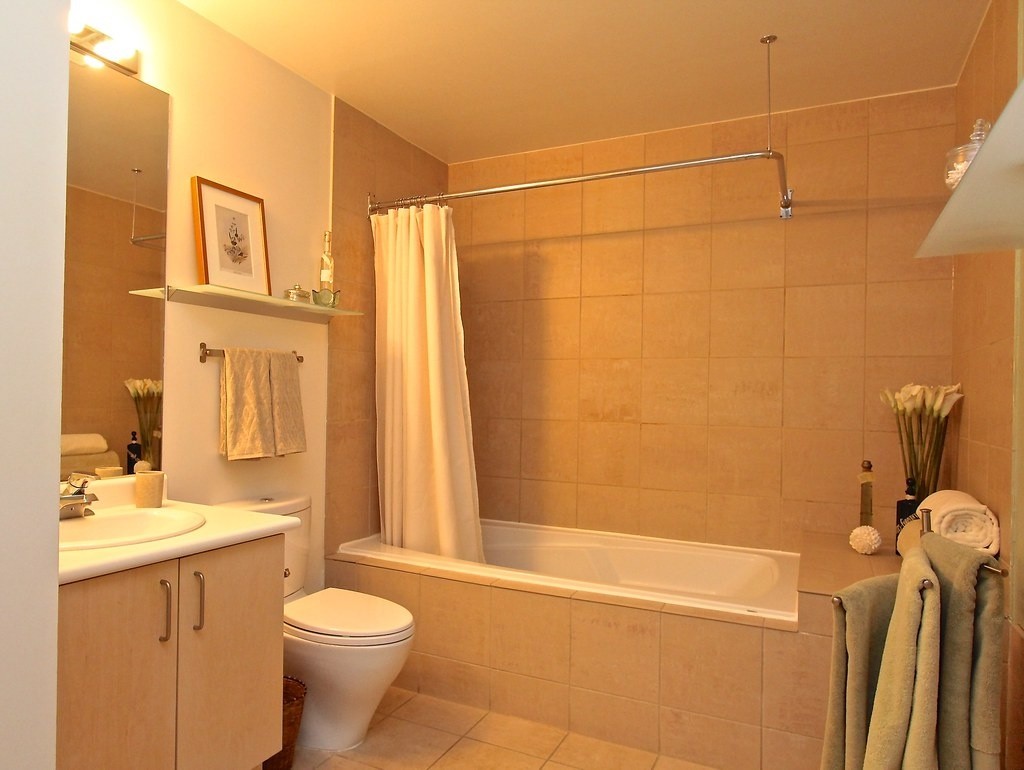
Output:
[61,433,108,456]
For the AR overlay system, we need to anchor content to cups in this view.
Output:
[95,467,123,476]
[136,471,164,508]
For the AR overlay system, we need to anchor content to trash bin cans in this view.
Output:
[262,676,307,770]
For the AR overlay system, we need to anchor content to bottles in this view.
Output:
[127,431,142,474]
[896,478,919,556]
[320,232,334,291]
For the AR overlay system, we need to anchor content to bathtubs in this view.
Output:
[334,514,801,770]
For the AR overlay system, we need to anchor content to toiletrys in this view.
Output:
[126,430,143,475]
[894,479,919,555]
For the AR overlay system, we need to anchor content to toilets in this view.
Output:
[212,490,416,751]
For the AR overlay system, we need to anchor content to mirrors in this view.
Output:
[62,51,170,480]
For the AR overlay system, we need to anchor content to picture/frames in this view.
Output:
[191,177,273,296]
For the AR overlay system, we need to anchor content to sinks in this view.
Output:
[59,508,206,552]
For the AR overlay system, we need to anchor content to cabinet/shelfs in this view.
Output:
[58,534,282,770]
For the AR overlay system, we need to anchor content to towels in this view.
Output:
[916,488,1000,557]
[819,535,1004,770]
[271,349,308,457]
[218,347,274,463]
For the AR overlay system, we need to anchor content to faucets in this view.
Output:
[59,467,101,520]
